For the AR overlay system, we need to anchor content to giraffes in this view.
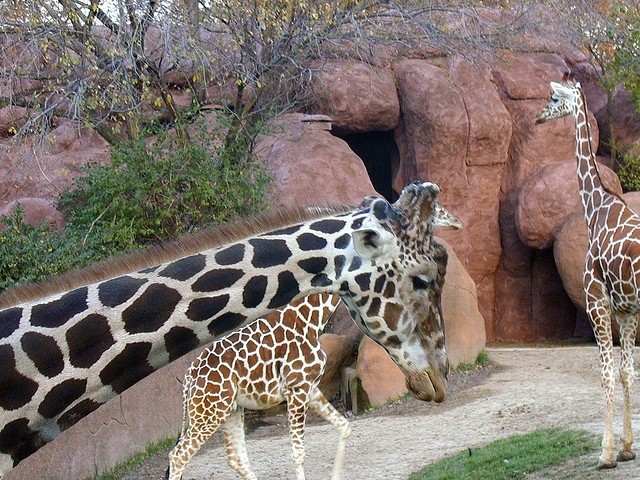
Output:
[166,202,463,480]
[0,181,449,480]
[535,71,640,471]
